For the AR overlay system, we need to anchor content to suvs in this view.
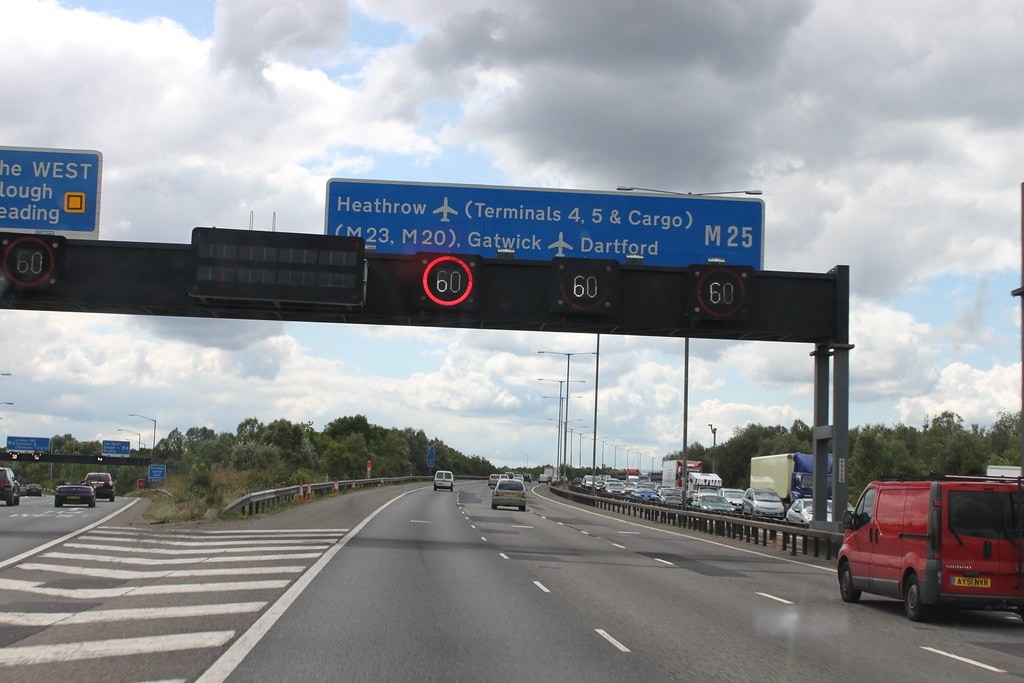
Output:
[0,467,23,506]
[835,479,1024,623]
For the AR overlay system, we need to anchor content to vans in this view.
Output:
[433,470,454,492]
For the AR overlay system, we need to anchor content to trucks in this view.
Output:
[749,452,835,503]
[688,472,724,497]
[662,461,702,491]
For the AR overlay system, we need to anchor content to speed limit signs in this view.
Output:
[416,252,492,323]
[687,263,752,327]
[552,255,620,322]
[0,233,75,304]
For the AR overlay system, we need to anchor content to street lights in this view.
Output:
[615,183,765,510]
[537,348,601,490]
[708,423,717,473]
[117,428,141,467]
[129,413,157,464]
[593,438,614,469]
[537,378,586,488]
[574,430,595,469]
[607,442,628,468]
[555,424,588,468]
[547,418,583,486]
[619,448,638,470]
[542,396,583,486]
[630,451,650,469]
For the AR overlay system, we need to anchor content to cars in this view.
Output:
[490,479,529,511]
[570,469,736,517]
[742,488,785,521]
[82,473,116,502]
[718,487,747,516]
[488,472,532,488]
[785,498,856,531]
[55,483,97,508]
[539,474,548,484]
[25,484,42,496]
